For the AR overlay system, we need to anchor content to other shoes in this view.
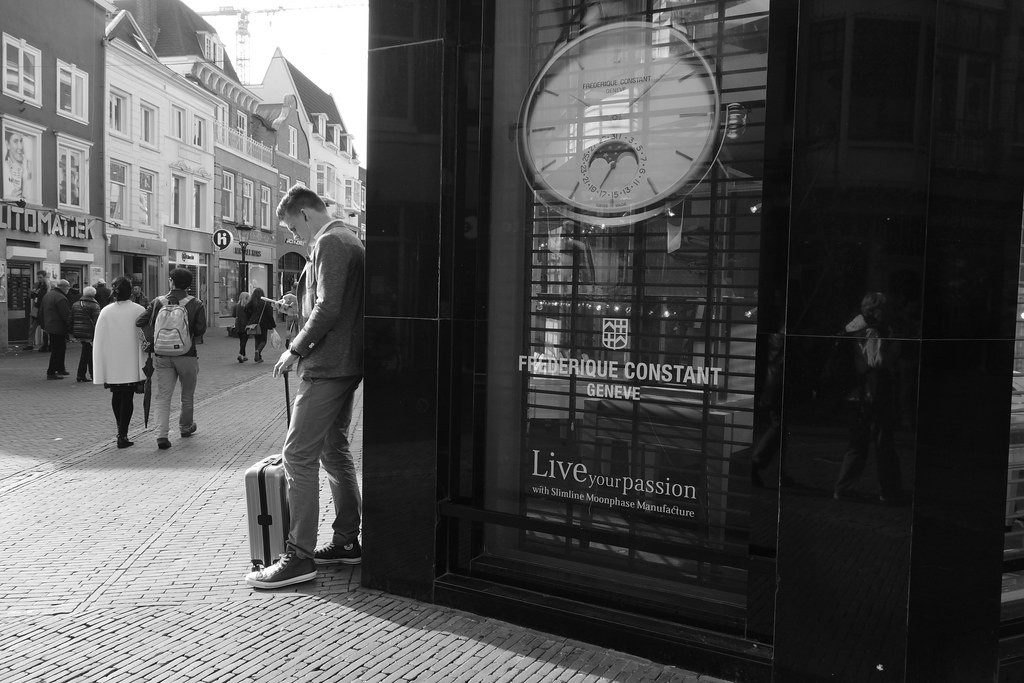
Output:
[117,435,133,448]
[181,423,196,437]
[76,377,91,382]
[59,371,70,375]
[254,350,260,362]
[237,354,244,362]
[243,357,249,361]
[22,345,32,350]
[38,345,50,352]
[67,338,73,342]
[157,438,171,448]
[47,374,63,380]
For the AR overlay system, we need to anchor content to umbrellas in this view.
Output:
[142,353,155,428]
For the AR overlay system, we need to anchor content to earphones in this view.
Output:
[305,215,307,222]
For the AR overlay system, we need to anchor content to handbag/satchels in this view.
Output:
[139,298,155,353]
[30,306,38,317]
[244,324,261,335]
[270,328,281,349]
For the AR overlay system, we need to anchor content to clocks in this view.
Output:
[518,19,740,227]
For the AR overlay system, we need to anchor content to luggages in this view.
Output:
[245,369,292,567]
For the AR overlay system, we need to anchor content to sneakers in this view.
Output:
[244,551,318,589]
[313,542,362,565]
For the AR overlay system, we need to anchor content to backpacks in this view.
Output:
[153,295,194,357]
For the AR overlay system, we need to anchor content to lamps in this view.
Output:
[349,213,355,217]
[0,199,26,207]
[96,217,122,229]
[49,210,71,222]
[326,203,329,207]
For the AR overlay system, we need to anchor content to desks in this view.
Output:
[584,392,754,552]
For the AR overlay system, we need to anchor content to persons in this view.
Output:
[200,277,207,307]
[237,287,276,362]
[740,288,910,508]
[136,268,206,449]
[131,285,150,309]
[92,277,146,448]
[22,269,120,381]
[283,291,300,338]
[247,185,366,588]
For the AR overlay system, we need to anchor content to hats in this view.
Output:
[169,268,193,282]
[98,280,105,284]
[131,278,143,287]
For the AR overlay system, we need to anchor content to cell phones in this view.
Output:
[261,297,290,307]
